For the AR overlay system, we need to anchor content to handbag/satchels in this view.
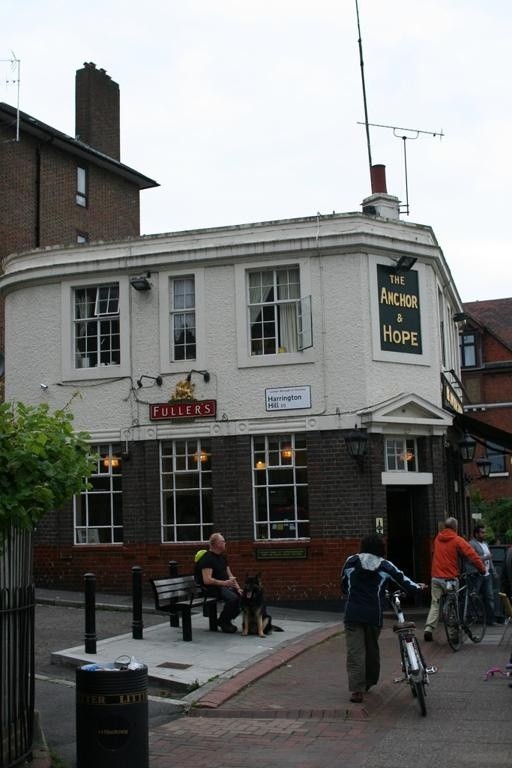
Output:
[193,549,207,583]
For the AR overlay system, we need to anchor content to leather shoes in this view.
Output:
[350,691,364,702]
[217,616,237,634]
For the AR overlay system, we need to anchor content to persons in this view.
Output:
[488,538,500,545]
[339,534,425,702]
[424,517,486,641]
[195,533,243,633]
[465,524,500,626]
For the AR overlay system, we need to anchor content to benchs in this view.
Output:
[148,571,224,642]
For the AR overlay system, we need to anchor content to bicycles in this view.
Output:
[439,570,488,652]
[379,581,438,715]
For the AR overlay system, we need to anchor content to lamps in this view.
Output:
[456,424,477,462]
[136,373,163,391]
[102,440,295,470]
[186,368,210,384]
[476,449,493,478]
[128,272,154,292]
[387,254,419,277]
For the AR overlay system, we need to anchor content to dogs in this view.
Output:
[237,570,286,638]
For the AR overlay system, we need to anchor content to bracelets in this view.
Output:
[236,588,241,591]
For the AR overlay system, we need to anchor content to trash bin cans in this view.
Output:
[75,662,150,768]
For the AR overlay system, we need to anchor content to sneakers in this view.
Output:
[424,627,433,641]
